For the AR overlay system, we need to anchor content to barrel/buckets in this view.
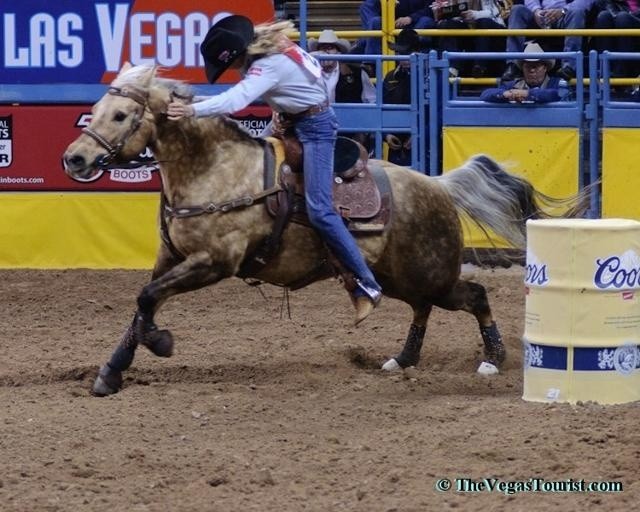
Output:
[522,218,640,407]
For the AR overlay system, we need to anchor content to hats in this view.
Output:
[308,30,350,52]
[514,43,555,71]
[200,15,253,85]
[388,30,428,52]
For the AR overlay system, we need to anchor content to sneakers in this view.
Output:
[501,64,517,80]
[472,64,484,77]
[449,67,460,76]
[557,67,575,80]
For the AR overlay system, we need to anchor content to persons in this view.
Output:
[307,0,640,167]
[166,14,382,325]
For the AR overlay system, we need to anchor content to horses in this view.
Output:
[59,61,607,398]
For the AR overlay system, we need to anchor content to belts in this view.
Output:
[282,100,329,120]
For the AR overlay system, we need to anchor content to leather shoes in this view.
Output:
[354,290,384,325]
